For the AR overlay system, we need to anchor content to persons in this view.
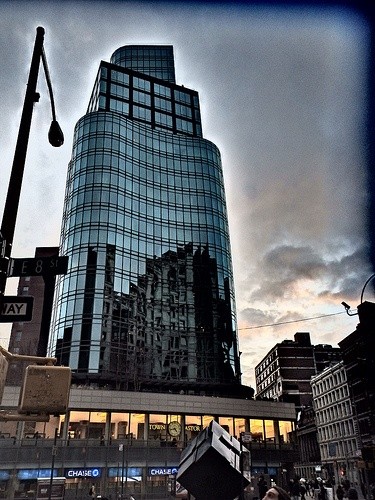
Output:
[291,473,375,500]
[91,482,96,500]
[258,476,268,499]
[261,486,290,500]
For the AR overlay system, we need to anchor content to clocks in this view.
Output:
[167,421,181,437]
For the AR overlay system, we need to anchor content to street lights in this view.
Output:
[1,26,65,289]
